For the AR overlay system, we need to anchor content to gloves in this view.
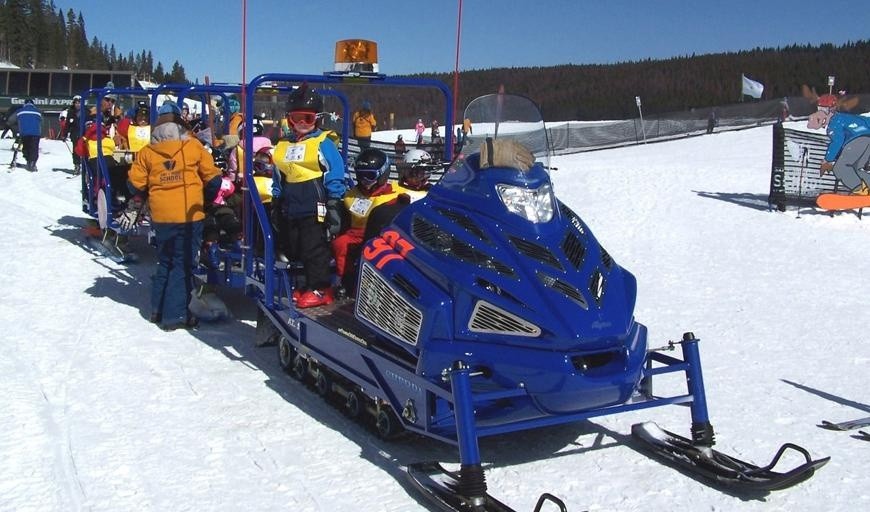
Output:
[479,140,536,172]
[62,136,67,142]
[119,200,143,232]
[270,197,281,234]
[323,199,351,235]
[820,159,833,177]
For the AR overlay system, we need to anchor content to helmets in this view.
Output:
[398,135,402,140]
[134,101,150,123]
[163,100,177,105]
[253,147,275,177]
[182,102,189,110]
[72,95,82,103]
[24,99,33,104]
[211,148,226,161]
[354,148,391,193]
[783,97,788,102]
[396,149,440,188]
[285,86,323,113]
[418,119,422,123]
[189,119,206,133]
[236,118,265,141]
[362,103,370,111]
[817,94,837,108]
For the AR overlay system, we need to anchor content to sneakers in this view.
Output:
[73,164,80,175]
[849,179,869,195]
[296,288,333,308]
[27,160,30,168]
[208,243,220,269]
[164,317,199,332]
[230,240,242,262]
[29,161,38,172]
[336,277,354,299]
[151,312,162,323]
[292,290,306,302]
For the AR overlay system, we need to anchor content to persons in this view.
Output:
[228,115,290,261]
[333,150,411,293]
[59,116,67,141]
[432,119,441,143]
[117,101,154,203]
[416,118,425,142]
[126,101,235,331]
[90,82,123,150]
[416,138,425,150]
[6,99,42,172]
[779,97,790,122]
[271,86,346,308]
[59,95,90,176]
[433,134,441,143]
[807,110,870,195]
[55,107,70,139]
[707,111,717,133]
[75,114,122,209]
[462,117,472,143]
[394,134,410,185]
[352,101,377,151]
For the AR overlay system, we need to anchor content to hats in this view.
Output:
[102,82,118,101]
[158,103,182,116]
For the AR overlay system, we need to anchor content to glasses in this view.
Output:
[286,111,316,126]
[215,162,228,171]
[817,105,830,115]
[104,97,116,103]
[356,155,389,181]
